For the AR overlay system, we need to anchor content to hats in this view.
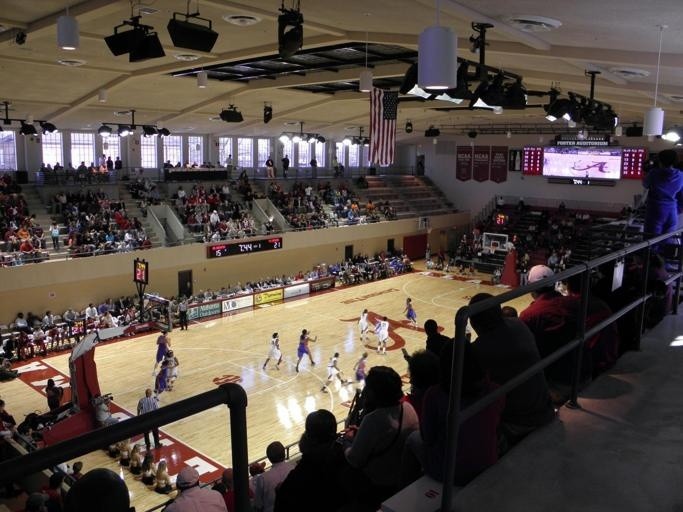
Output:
[177,466,199,487]
[528,264,555,287]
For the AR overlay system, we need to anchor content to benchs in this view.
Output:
[453,203,625,276]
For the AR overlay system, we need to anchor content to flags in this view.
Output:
[366,86,399,169]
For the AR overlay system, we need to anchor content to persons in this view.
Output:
[1,153,397,268]
[1,250,672,512]
[642,150,683,249]
[397,200,588,271]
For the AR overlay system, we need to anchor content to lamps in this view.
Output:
[405,0,669,137]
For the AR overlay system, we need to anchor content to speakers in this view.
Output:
[626,127,643,136]
[103,12,217,63]
[425,128,440,137]
[220,108,243,122]
